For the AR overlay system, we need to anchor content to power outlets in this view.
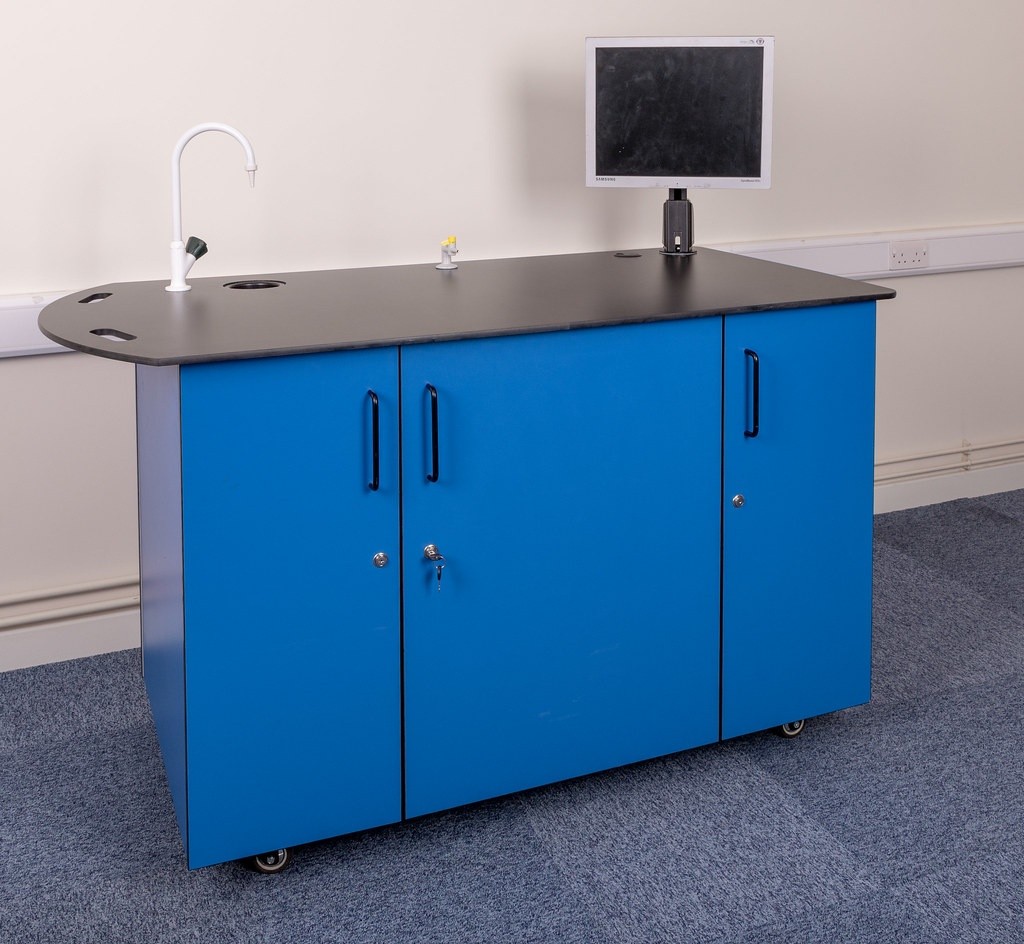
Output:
[885,240,926,271]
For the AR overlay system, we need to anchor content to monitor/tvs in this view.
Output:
[585,35,771,190]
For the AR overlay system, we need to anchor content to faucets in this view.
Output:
[165,122,259,292]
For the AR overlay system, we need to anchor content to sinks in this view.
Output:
[222,279,287,290]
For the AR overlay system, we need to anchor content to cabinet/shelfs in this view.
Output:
[134,301,877,871]
